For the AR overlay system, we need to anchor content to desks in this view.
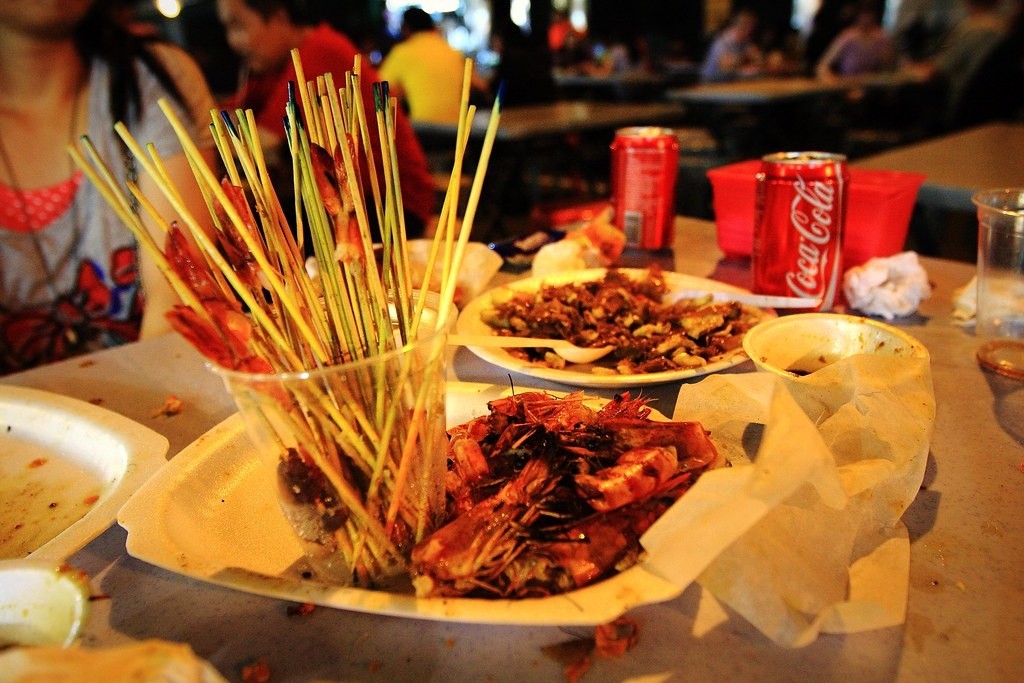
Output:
[850,124,1024,209]
[669,75,851,155]
[473,100,684,242]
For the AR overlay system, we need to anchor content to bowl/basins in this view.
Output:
[742,312,930,389]
[0,561,87,651]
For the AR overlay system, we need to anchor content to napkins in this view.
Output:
[638,355,937,648]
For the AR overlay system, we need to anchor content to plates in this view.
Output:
[116,379,688,626]
[0,385,169,565]
[456,268,779,388]
[406,239,503,310]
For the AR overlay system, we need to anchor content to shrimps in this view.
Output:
[410,389,729,597]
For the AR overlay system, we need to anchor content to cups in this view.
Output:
[970,187,1024,381]
[206,290,458,589]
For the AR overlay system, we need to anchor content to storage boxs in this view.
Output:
[706,160,926,269]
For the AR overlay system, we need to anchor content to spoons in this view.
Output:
[448,334,616,364]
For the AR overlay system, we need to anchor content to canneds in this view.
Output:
[751,152,846,316]
[608,126,679,253]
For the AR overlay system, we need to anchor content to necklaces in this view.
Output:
[0,74,93,343]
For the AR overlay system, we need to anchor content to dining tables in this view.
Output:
[0,216,1024,683]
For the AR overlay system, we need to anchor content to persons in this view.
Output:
[216,0,430,312]
[0,0,216,377]
[374,0,1024,174]
